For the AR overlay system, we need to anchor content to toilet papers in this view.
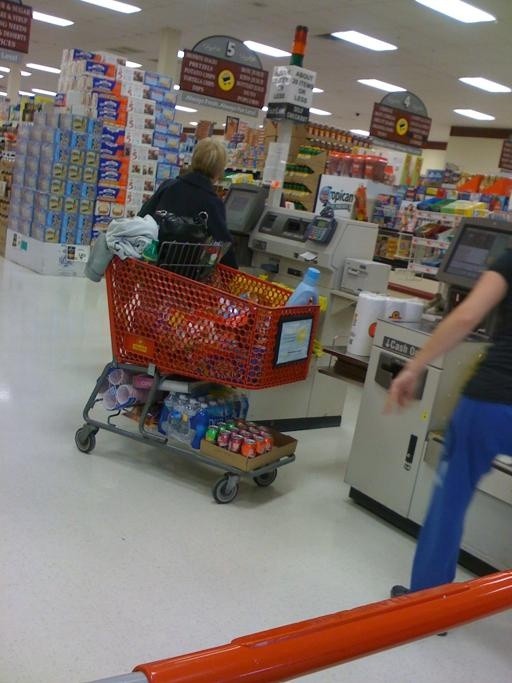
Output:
[347,290,386,359]
[403,297,425,320]
[380,295,408,321]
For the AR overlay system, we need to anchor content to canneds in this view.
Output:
[205,419,274,458]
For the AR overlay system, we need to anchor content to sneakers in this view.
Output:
[390,585,449,638]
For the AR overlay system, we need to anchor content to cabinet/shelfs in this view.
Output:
[405,210,465,283]
[0,118,26,256]
[226,138,327,212]
[343,319,512,574]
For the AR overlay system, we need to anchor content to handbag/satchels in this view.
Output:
[153,210,208,262]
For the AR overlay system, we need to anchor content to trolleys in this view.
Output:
[76,228,320,499]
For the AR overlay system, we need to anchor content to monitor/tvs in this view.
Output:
[434,217,511,291]
[223,182,267,235]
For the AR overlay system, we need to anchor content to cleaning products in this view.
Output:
[283,266,321,308]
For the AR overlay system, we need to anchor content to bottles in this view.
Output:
[279,120,374,211]
[158,386,249,453]
[141,239,160,266]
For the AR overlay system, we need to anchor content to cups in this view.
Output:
[102,368,152,410]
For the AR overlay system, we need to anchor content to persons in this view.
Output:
[136,137,239,271]
[382,234,510,637]
